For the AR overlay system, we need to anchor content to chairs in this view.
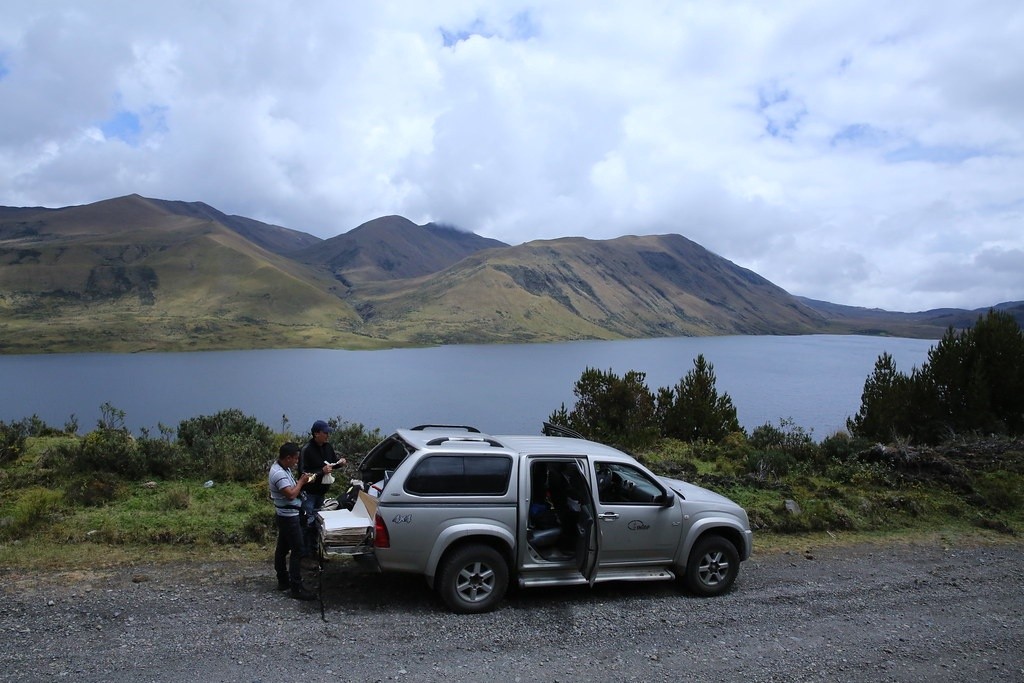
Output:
[526,471,594,550]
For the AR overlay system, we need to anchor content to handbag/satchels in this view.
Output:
[277,505,305,519]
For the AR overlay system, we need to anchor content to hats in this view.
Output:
[311,420,334,433]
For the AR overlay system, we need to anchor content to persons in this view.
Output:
[269,441,314,602]
[298,419,347,572]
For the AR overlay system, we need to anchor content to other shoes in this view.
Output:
[289,587,317,600]
[278,579,291,590]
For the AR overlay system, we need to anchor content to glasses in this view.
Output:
[324,431,329,434]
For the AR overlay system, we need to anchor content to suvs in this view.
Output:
[336,422,751,614]
